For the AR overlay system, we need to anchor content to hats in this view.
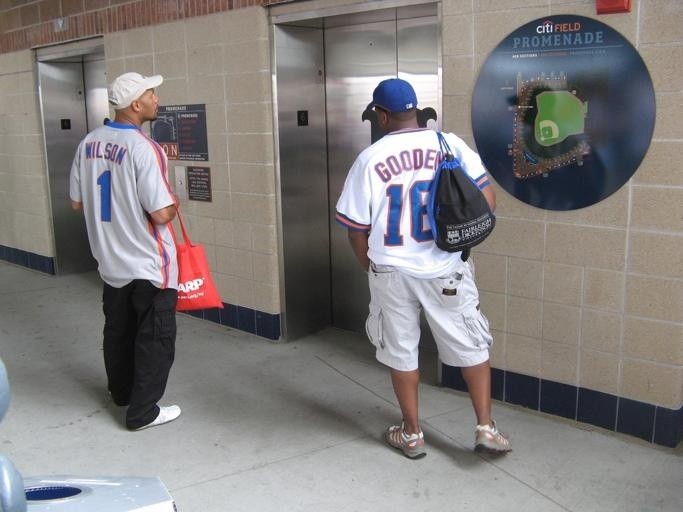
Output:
[367,79,417,113]
[108,72,163,110]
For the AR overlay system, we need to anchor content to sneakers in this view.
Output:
[133,405,181,430]
[385,421,426,459]
[475,419,512,457]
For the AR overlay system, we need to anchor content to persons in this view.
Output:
[70,70,181,430]
[333,78,513,458]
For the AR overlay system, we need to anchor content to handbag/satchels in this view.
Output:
[176,243,224,311]
[426,159,496,251]
